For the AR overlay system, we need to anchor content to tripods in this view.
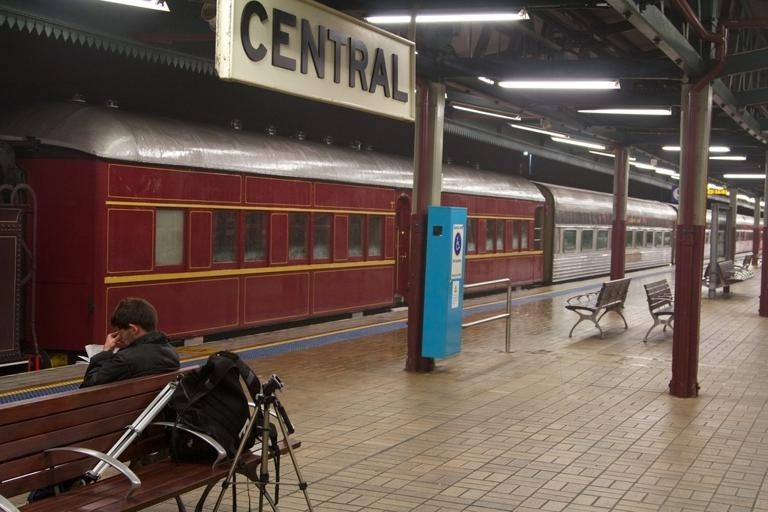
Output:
[211,394,315,512]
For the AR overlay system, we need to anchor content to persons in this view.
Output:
[17,296,181,505]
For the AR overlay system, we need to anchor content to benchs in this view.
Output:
[0,364,303,512]
[702,261,754,298]
[565,277,674,343]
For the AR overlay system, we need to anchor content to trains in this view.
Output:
[0,102,766,375]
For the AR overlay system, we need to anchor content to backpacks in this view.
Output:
[165,349,261,464]
